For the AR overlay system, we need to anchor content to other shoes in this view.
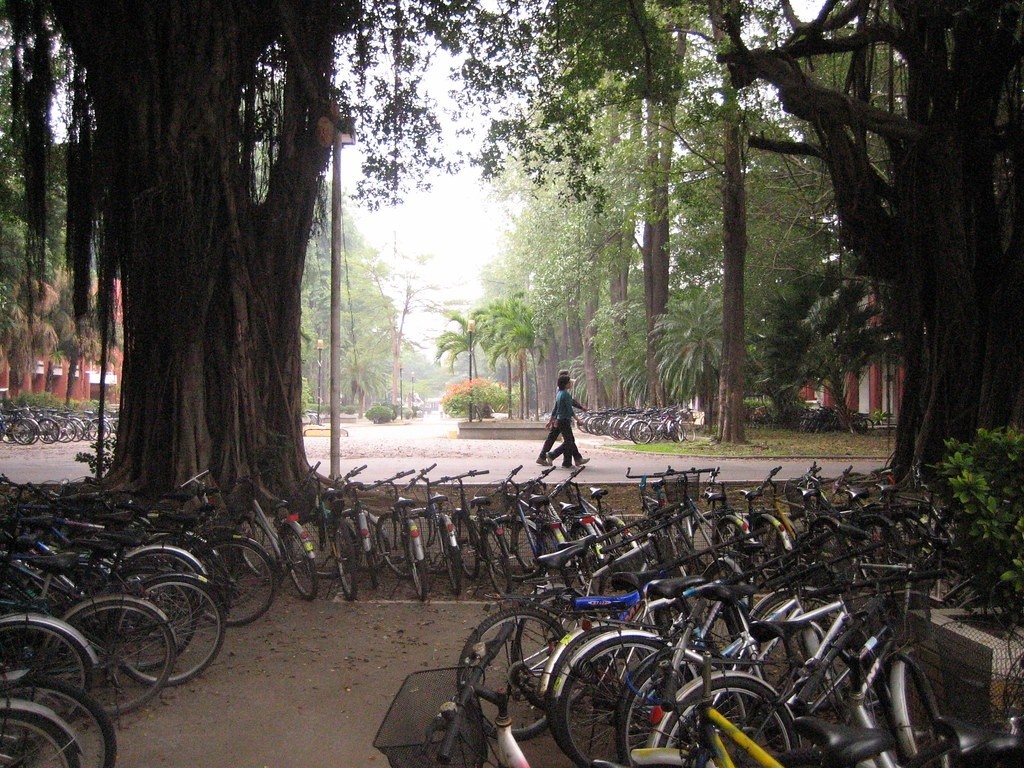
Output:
[562,462,575,469]
[546,452,552,466]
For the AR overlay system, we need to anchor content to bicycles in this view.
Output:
[0,401,119,445]
[0,450,1024,768]
[303,408,323,427]
[574,403,696,445]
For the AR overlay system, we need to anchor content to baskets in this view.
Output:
[845,587,931,649]
[276,479,318,526]
[784,476,806,516]
[220,478,264,525]
[933,620,1024,732]
[373,666,487,768]
[471,485,505,518]
[662,474,700,503]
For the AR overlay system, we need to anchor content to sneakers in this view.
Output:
[575,458,591,466]
[536,457,550,465]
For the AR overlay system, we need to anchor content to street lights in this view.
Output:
[316,339,325,426]
[399,360,403,421]
[466,318,476,422]
[410,372,415,410]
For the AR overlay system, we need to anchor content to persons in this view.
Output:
[536,369,591,470]
